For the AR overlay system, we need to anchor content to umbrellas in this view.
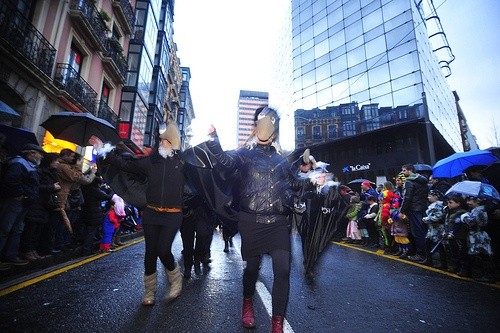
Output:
[39,111,120,156]
[413,162,432,175]
[431,150,500,179]
[0,101,21,119]
[443,180,500,203]
[0,123,38,156]
[346,177,375,193]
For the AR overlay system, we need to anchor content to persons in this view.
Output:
[0,138,152,265]
[207,105,293,333]
[178,201,240,279]
[88,119,219,305]
[293,154,500,282]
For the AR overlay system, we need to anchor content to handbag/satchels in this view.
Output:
[46,188,64,209]
[68,189,85,208]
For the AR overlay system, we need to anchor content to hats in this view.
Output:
[22,144,45,153]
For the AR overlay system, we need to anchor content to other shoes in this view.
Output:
[194,262,201,274]
[23,250,45,260]
[224,245,229,252]
[418,260,434,266]
[229,238,233,247]
[0,252,29,272]
[99,249,112,253]
[40,249,61,255]
[202,262,210,271]
[407,253,425,261]
[184,266,191,278]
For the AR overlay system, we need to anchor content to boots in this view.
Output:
[272,315,284,333]
[242,297,255,328]
[163,261,183,297]
[111,233,119,246]
[393,245,411,259]
[142,270,158,305]
[114,233,125,245]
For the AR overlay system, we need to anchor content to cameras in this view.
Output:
[89,166,95,170]
